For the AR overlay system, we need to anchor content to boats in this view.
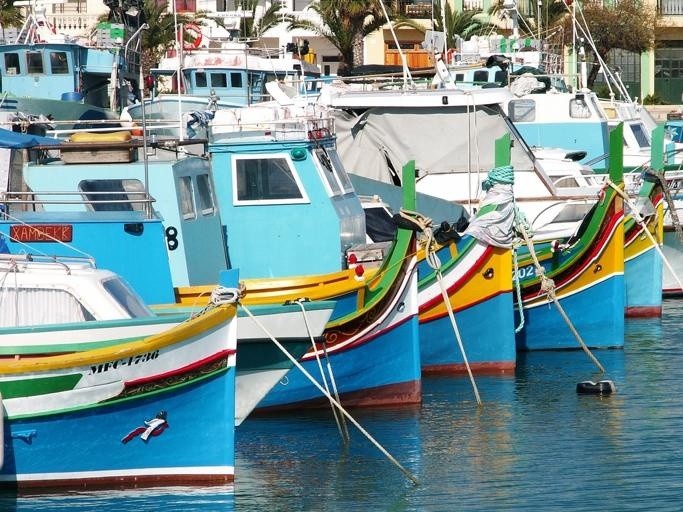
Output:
[0,0,682,495]
